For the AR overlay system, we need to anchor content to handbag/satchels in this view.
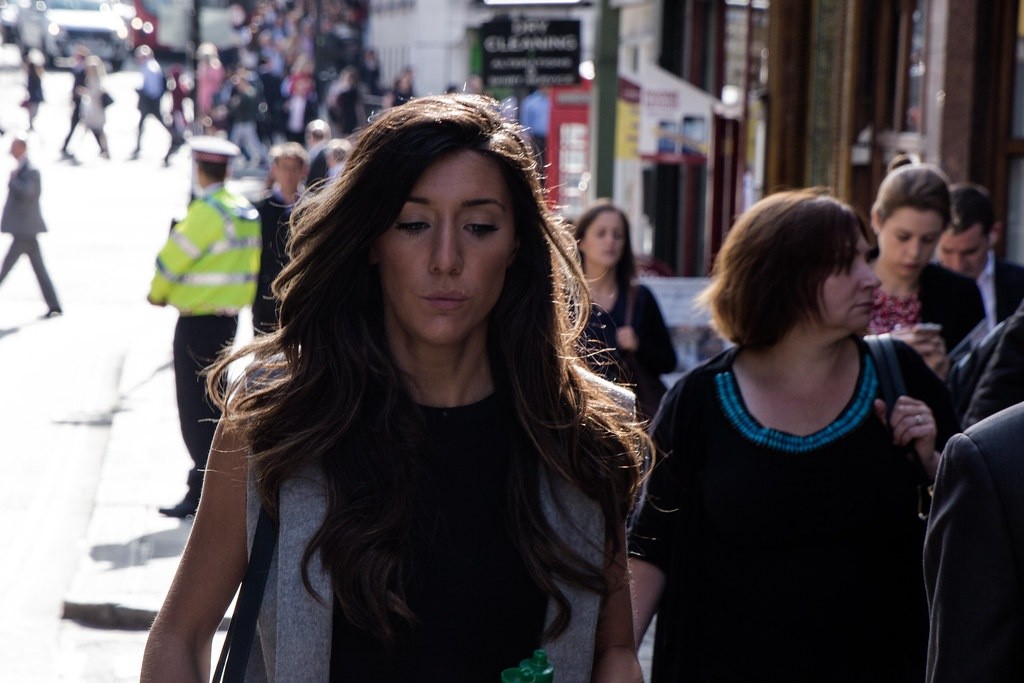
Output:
[617,361,670,417]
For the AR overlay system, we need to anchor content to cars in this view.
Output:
[0,0,21,45]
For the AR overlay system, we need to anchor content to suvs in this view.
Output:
[16,0,131,71]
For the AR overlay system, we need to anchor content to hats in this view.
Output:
[185,136,241,163]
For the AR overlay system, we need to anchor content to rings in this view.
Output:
[915,415,923,424]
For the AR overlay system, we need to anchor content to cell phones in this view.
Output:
[912,324,943,342]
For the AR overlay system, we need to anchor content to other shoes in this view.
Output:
[159,487,199,515]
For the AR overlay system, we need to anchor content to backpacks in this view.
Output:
[944,316,1006,415]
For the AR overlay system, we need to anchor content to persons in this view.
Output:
[147,119,352,517]
[627,188,962,683]
[20,63,44,132]
[870,149,1024,683]
[129,0,414,168]
[140,94,644,683]
[518,85,550,187]
[61,44,114,159]
[0,137,62,319]
[445,74,484,94]
[566,204,678,422]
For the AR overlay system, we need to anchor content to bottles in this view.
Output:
[500,648,556,683]
